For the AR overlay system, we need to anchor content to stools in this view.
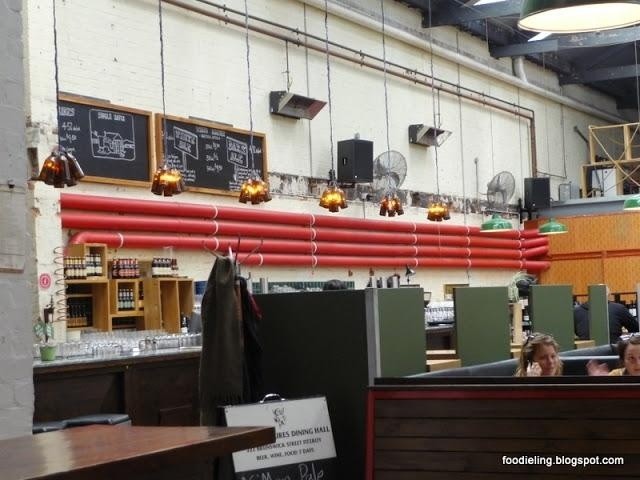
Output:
[32,413,132,434]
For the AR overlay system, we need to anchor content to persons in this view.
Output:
[585,332,640,376]
[573,283,639,345]
[513,331,566,377]
[322,278,345,291]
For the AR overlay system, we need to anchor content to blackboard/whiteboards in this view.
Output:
[57,91,157,188]
[155,112,270,199]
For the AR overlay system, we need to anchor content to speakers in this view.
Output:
[524,177,550,210]
[337,140,373,183]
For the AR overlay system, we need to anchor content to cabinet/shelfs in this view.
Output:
[63,243,195,333]
[573,291,638,332]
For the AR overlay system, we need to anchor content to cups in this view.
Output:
[34,327,202,362]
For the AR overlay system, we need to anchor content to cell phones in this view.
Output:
[525,352,533,366]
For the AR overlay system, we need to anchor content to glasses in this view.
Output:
[618,331,640,341]
[525,335,536,346]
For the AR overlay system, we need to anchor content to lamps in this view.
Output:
[517,0,640,34]
[39,0,450,221]
[479,193,640,236]
[404,263,416,284]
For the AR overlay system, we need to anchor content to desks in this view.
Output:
[0,422,277,480]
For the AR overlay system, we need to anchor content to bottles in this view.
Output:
[62,252,190,333]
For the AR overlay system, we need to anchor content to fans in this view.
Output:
[486,170,515,213]
